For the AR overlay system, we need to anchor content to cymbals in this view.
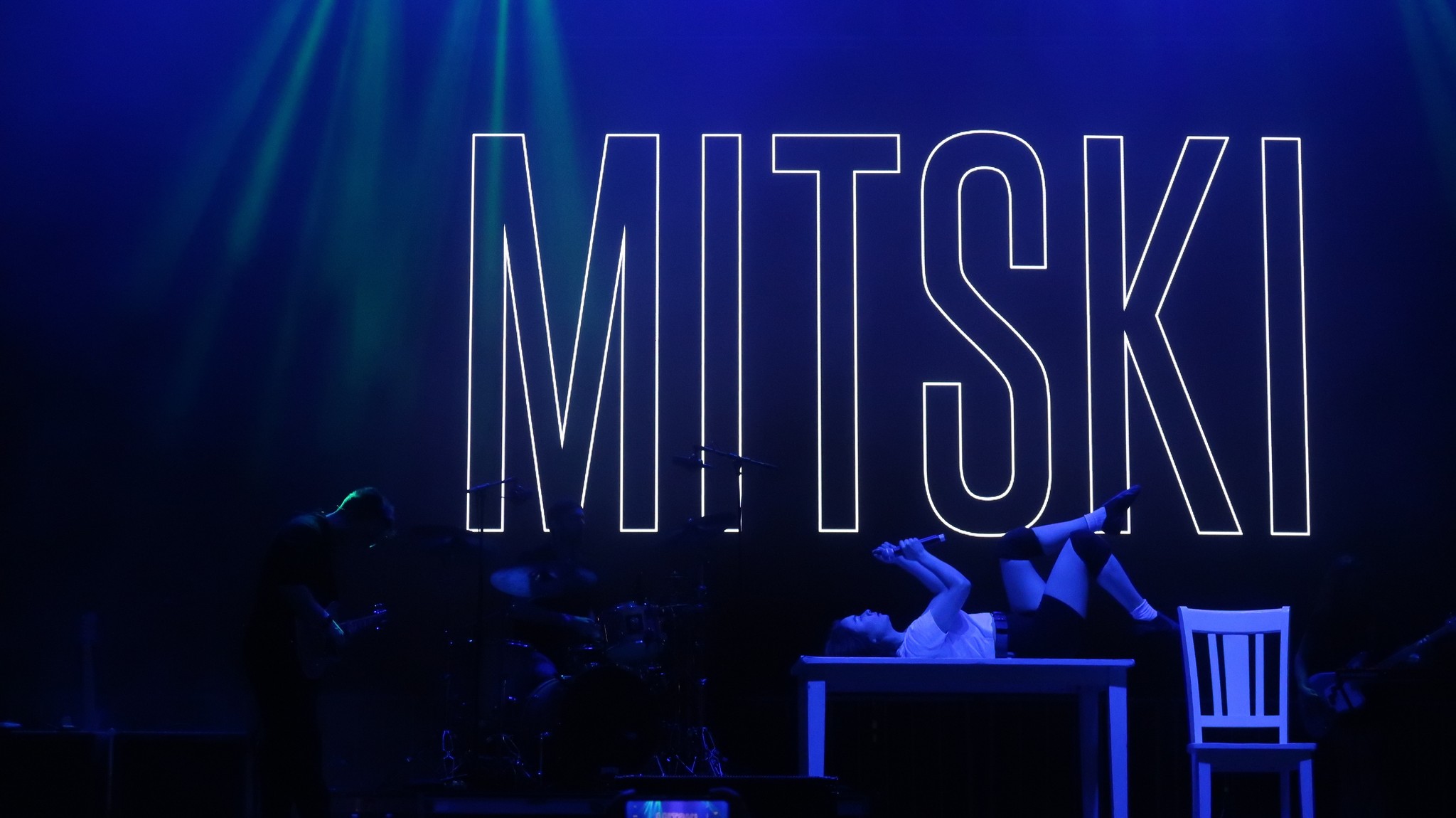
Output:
[662,513,736,550]
[487,560,600,598]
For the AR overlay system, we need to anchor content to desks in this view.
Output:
[795,655,1135,818]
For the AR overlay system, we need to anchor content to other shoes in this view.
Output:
[1133,611,1180,635]
[1102,484,1142,534]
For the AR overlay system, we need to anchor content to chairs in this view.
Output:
[1179,604,1319,818]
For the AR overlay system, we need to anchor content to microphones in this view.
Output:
[673,453,710,470]
[867,535,946,564]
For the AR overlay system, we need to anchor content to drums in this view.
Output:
[565,638,610,681]
[479,638,560,741]
[601,602,670,663]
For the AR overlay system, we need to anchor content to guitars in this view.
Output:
[296,603,394,682]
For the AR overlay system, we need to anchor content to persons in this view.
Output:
[241,487,391,818]
[532,502,664,686]
[819,485,1183,656]
